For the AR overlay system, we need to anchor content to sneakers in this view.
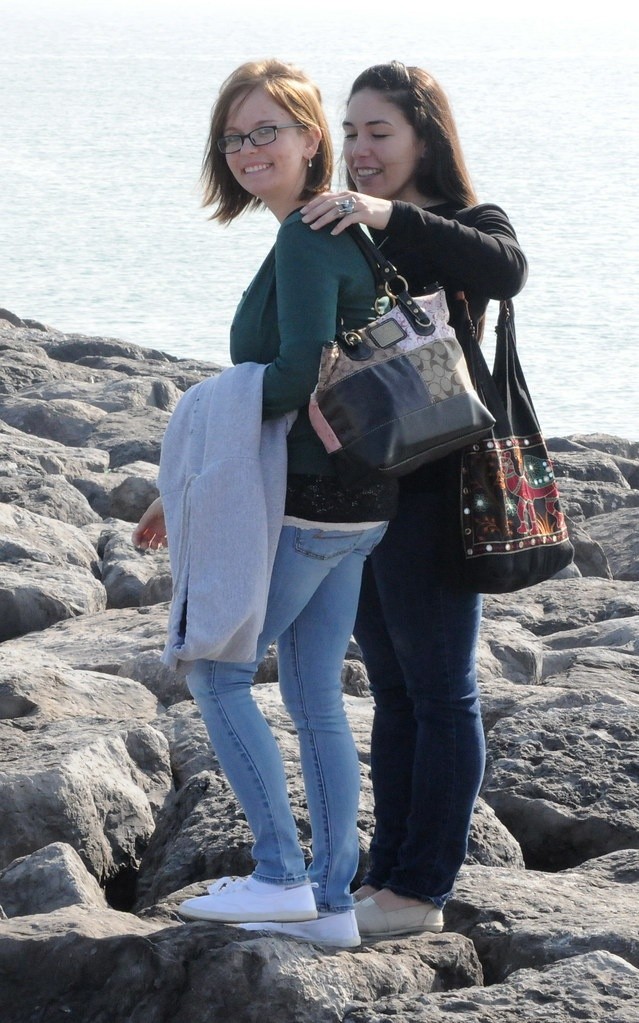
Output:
[179,875,318,924]
[249,907,362,947]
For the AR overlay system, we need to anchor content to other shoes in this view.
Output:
[351,892,445,936]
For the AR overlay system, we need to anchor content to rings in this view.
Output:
[333,196,357,217]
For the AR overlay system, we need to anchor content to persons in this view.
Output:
[300,61,529,937]
[131,57,401,948]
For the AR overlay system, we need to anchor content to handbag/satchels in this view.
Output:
[283,204,498,480]
[446,296,574,594]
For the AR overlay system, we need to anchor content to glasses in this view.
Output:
[217,123,306,155]
